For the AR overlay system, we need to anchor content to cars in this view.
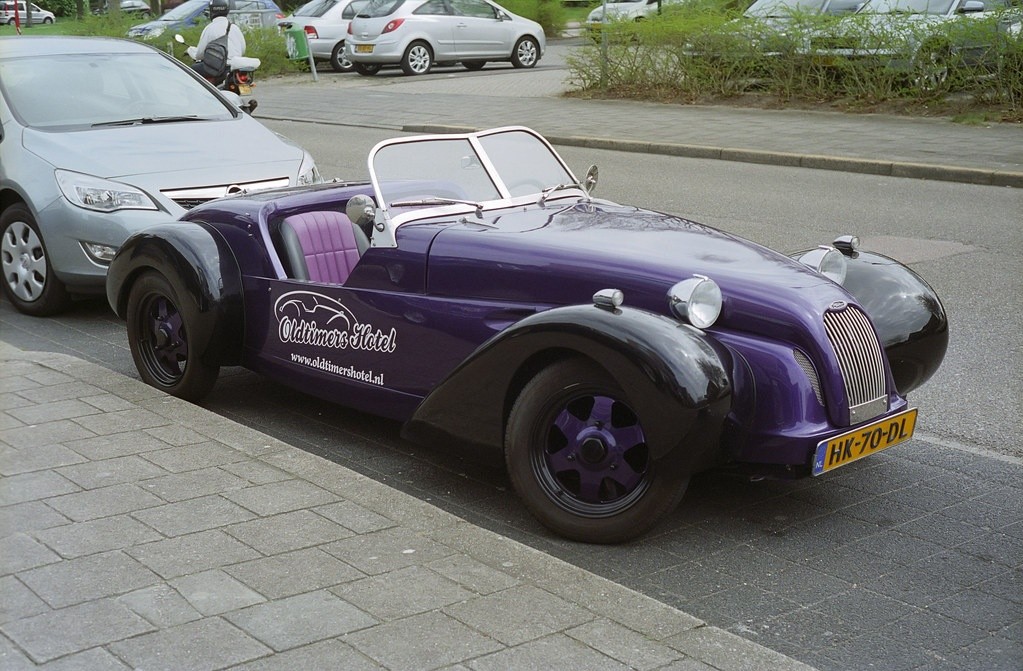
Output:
[586,0,684,44]
[712,1,1023,100]
[345,0,547,77]
[275,0,370,73]
[0,34,324,317]
[94,0,152,21]
[123,0,284,42]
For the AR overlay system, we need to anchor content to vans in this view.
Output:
[0,0,56,26]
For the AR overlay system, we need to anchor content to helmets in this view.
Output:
[209,0,229,20]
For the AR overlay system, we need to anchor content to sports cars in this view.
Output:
[106,126,953,549]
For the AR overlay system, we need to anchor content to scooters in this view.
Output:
[174,34,258,117]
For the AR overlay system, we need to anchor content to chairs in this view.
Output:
[277,193,451,286]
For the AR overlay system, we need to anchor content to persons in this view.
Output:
[184,0,247,86]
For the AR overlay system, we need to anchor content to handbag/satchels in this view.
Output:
[199,19,232,77]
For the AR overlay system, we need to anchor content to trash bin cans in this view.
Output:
[285,23,310,62]
